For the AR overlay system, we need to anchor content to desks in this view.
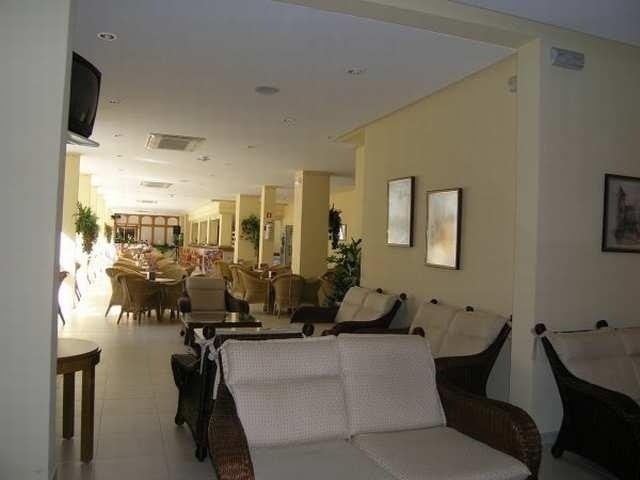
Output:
[185,311,305,356]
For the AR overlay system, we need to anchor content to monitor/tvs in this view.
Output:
[68,50,102,138]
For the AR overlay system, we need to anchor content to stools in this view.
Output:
[56,334,102,464]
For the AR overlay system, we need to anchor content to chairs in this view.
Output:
[106,240,322,321]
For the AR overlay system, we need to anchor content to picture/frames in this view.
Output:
[424,185,464,270]
[598,173,639,255]
[383,176,415,248]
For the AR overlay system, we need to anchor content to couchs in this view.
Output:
[171,322,425,461]
[176,274,248,344]
[210,328,543,480]
[290,284,513,392]
[533,319,638,479]
